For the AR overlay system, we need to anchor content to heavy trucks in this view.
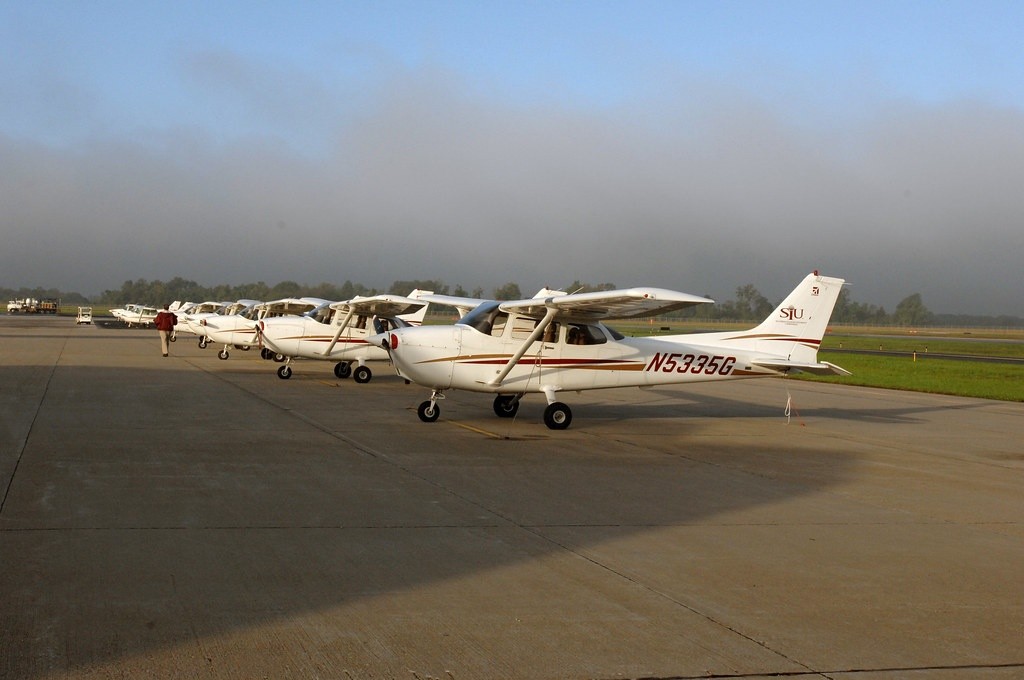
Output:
[7,298,58,315]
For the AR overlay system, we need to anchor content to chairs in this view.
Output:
[568,328,588,345]
[324,317,330,324]
[381,320,388,332]
[477,320,491,336]
[315,315,324,322]
[534,321,544,341]
[542,323,557,343]
[354,316,366,329]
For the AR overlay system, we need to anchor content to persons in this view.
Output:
[153,304,178,356]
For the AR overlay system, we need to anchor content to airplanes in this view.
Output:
[109,289,511,385]
[366,269,855,433]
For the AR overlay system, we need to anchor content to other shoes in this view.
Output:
[163,353,168,357]
